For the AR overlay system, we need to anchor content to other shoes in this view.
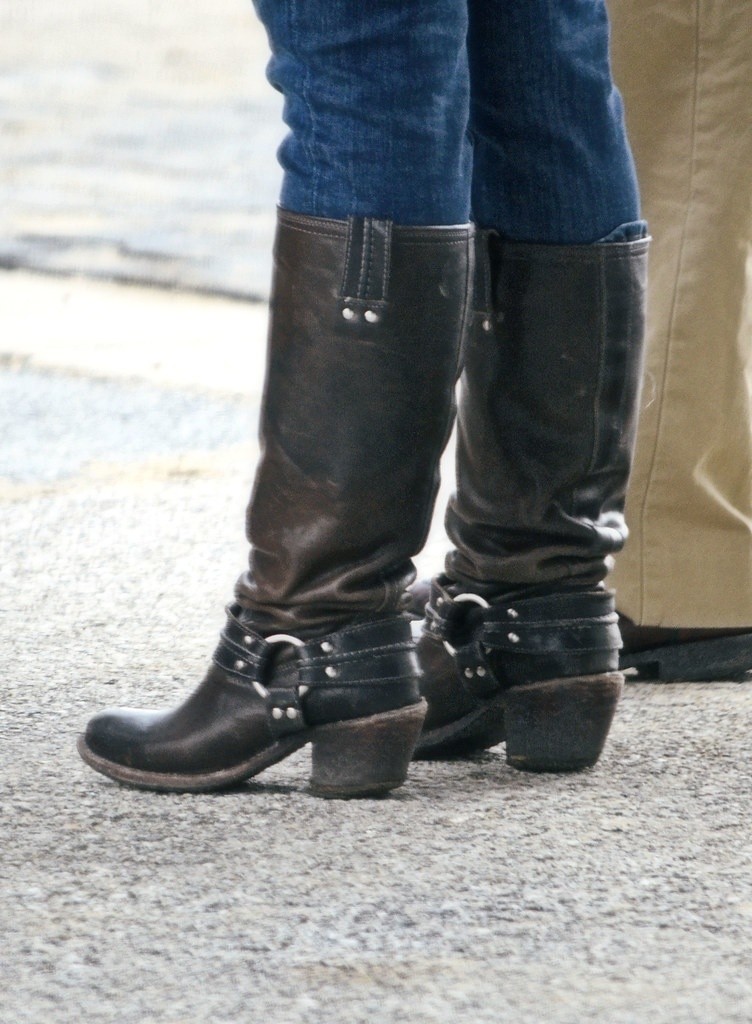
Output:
[609,609,752,682]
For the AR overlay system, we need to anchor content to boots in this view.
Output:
[400,230,652,773]
[76,210,476,802]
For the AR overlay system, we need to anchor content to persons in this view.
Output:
[78,0,651,796]
